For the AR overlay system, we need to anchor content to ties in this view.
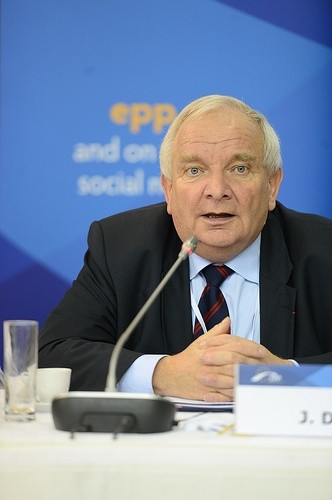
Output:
[193,265,235,340]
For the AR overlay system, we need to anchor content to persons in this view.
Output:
[38,95,332,402]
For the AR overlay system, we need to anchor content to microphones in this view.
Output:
[52,235,200,434]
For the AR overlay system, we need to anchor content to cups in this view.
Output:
[37,367,71,411]
[2,320,38,423]
